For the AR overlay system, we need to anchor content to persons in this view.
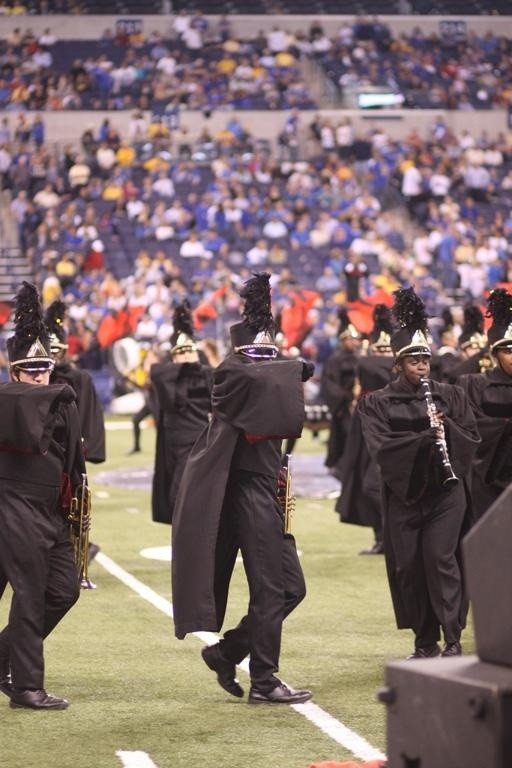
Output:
[200,348,315,705]
[1,0,512,557]
[0,369,91,710]
[355,354,481,660]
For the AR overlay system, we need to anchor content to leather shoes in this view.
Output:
[404,641,462,660]
[1,673,69,710]
[360,541,383,555]
[202,639,243,698]
[248,681,313,704]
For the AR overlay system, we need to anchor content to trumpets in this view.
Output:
[67,473,97,591]
[275,453,302,557]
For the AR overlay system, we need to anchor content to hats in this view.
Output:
[338,321,512,364]
[229,319,281,359]
[159,331,197,355]
[5,323,69,372]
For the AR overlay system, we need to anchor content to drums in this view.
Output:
[113,336,142,375]
